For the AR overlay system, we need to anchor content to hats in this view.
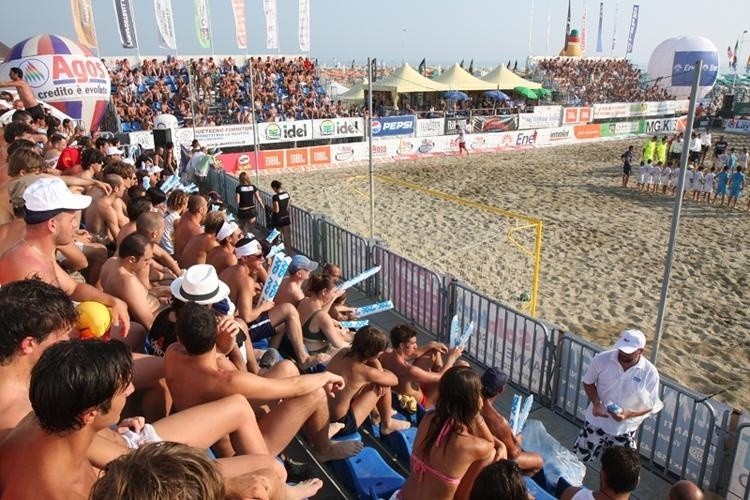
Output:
[170,263,232,305]
[483,366,508,389]
[22,177,93,212]
[106,146,124,156]
[6,176,45,208]
[148,165,163,176]
[614,328,647,354]
[288,255,319,274]
[74,125,86,132]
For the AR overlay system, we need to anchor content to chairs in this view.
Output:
[139,84,146,91]
[331,431,362,444]
[331,447,406,499]
[390,427,433,471]
[516,474,560,500]
[366,407,408,436]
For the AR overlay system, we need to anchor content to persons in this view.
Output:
[1,55,749,498]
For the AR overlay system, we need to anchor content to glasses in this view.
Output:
[234,231,243,238]
[27,119,35,124]
[246,252,265,260]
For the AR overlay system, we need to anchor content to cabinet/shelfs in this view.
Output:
[132,121,142,130]
[122,120,131,133]
